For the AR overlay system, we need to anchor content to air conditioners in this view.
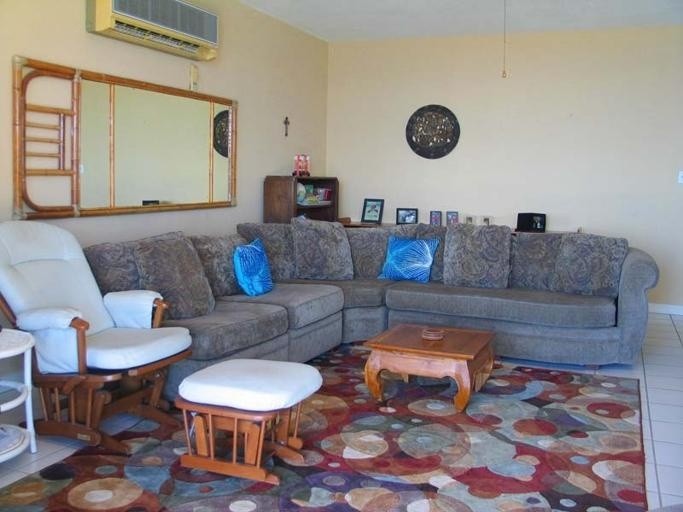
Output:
[85,0,220,63]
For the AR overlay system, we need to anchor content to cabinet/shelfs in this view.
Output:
[263,175,341,226]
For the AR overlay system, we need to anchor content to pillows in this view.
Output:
[133,218,274,319]
[374,222,629,299]
[289,216,355,282]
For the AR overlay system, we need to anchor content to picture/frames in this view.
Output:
[360,198,459,226]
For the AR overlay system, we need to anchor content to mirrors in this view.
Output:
[73,67,236,215]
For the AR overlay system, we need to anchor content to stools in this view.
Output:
[172,355,326,488]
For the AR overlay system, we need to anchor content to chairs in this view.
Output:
[0,219,197,457]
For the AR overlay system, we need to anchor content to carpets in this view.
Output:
[0,340,649,512]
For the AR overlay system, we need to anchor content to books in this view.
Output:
[303,183,331,200]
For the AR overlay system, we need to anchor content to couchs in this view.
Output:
[77,229,660,417]
